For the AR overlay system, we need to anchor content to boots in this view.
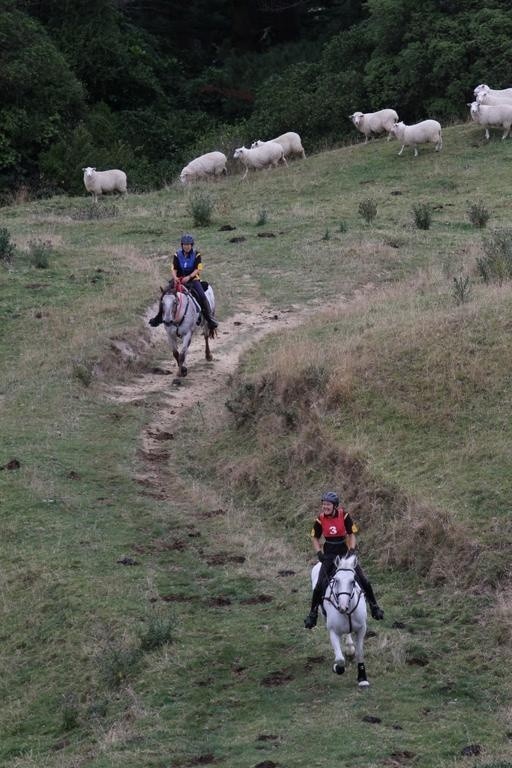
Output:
[364,582,384,620]
[302,589,321,628]
[149,302,165,327]
[201,300,219,328]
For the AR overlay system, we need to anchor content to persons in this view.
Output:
[304,491,384,629]
[149,235,218,329]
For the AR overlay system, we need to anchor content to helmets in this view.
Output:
[321,492,339,506]
[181,235,194,245]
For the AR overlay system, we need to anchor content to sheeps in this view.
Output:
[468,84,511,141]
[81,166,129,202]
[179,151,228,183]
[233,143,289,180]
[390,119,442,156]
[349,109,398,143]
[250,131,307,172]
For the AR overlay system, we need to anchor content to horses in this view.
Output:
[310,553,369,687]
[160,281,216,388]
